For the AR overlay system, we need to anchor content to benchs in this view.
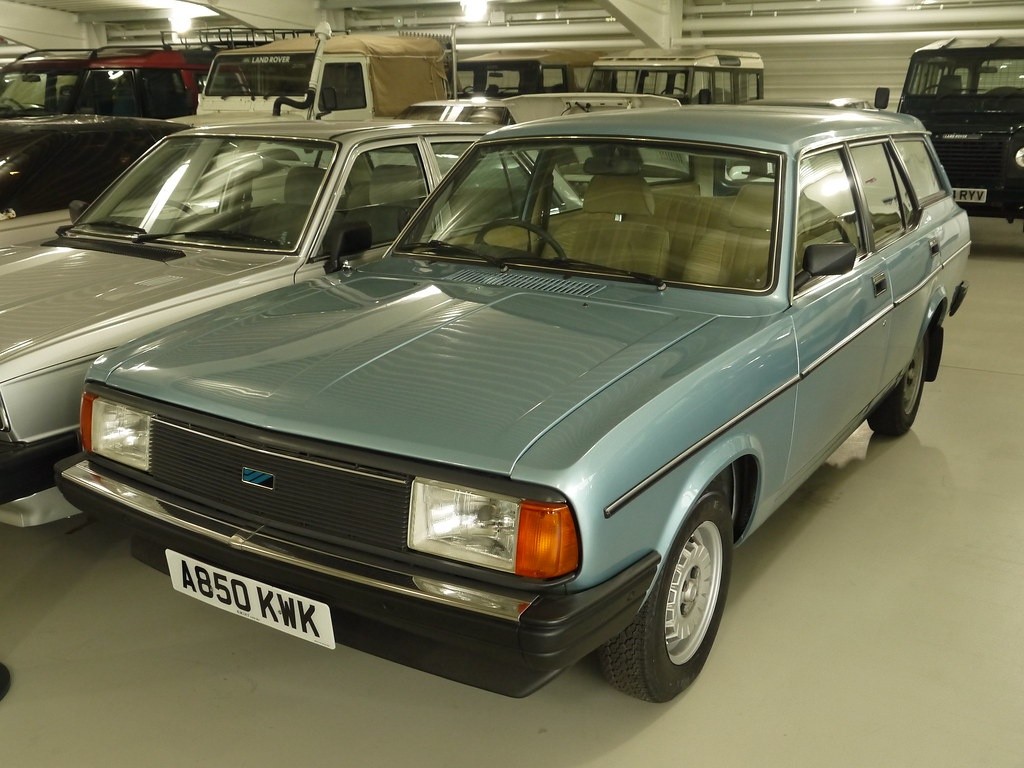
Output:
[344,182,545,233]
[655,195,867,280]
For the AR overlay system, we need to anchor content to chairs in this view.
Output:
[58,85,75,109]
[249,166,347,255]
[695,88,731,104]
[541,174,672,280]
[355,164,424,244]
[683,185,814,287]
[937,75,962,95]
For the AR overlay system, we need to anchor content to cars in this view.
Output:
[0,113,199,222]
[0,113,585,523]
[873,35,1024,226]
[46,101,975,703]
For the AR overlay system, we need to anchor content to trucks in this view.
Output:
[0,22,795,180]
